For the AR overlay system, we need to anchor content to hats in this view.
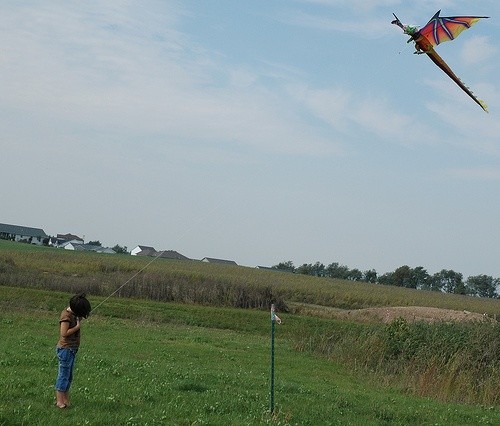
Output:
[70,295,91,315]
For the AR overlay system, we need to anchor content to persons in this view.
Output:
[55,292,91,409]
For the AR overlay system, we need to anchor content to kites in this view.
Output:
[391,9,491,113]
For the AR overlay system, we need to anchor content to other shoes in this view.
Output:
[57,401,65,408]
[64,401,70,407]
